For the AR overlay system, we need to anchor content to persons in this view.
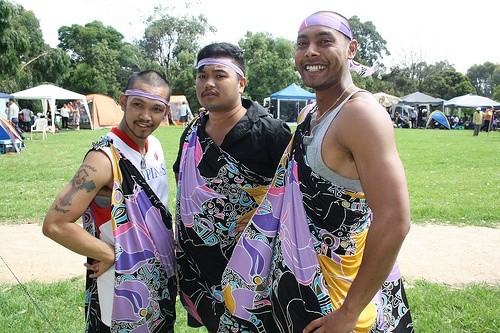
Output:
[179,100,190,129]
[389,111,411,129]
[287,103,302,122]
[59,101,81,131]
[452,113,473,128]
[268,105,276,115]
[473,107,483,137]
[174,43,292,333]
[410,109,416,128]
[42,70,175,332]
[218,12,415,333]
[5,98,35,141]
[416,107,422,128]
[484,107,492,132]
[47,107,53,134]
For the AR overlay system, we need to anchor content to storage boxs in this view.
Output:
[0,139,23,154]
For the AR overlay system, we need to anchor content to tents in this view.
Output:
[444,93,500,118]
[270,83,316,120]
[9,83,87,101]
[0,93,15,98]
[159,95,195,127]
[400,92,446,114]
[75,93,126,130]
[426,110,451,130]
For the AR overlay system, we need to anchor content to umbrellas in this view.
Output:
[371,92,402,107]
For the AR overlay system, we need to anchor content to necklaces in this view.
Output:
[315,83,355,123]
[134,146,149,169]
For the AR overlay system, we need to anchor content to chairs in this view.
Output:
[31,119,49,139]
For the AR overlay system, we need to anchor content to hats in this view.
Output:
[475,107,481,110]
